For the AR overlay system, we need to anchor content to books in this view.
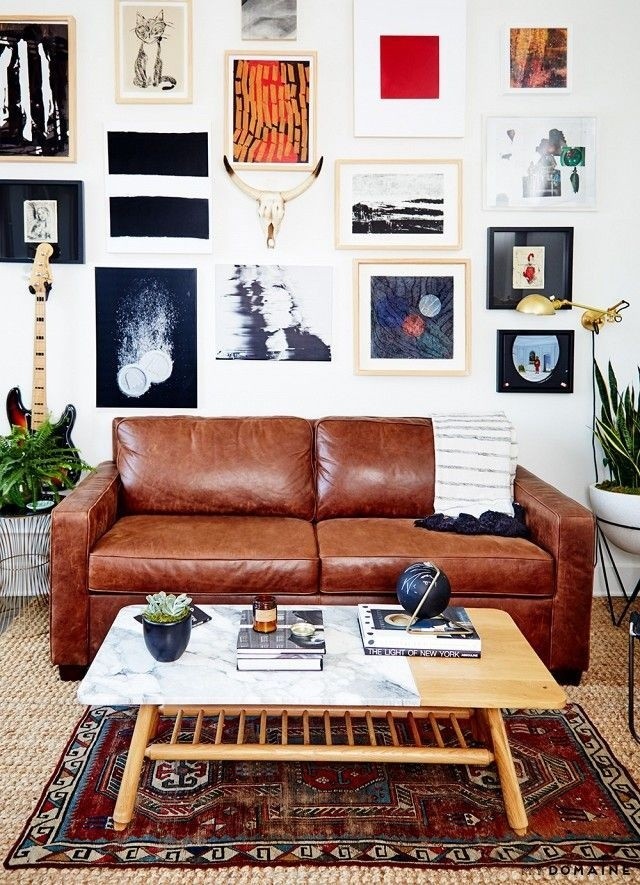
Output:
[236,610,327,671]
[357,603,482,658]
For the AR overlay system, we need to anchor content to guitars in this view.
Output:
[6,242,83,491]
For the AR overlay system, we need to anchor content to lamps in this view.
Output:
[515,294,630,330]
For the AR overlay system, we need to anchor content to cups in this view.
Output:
[252,596,277,633]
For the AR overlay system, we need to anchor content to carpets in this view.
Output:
[3,703,640,870]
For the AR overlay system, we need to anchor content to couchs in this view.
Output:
[51,416,594,684]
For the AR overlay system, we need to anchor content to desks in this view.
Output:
[78,604,566,836]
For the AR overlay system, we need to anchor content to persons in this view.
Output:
[25,202,54,239]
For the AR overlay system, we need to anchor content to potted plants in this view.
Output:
[586,357,640,555]
[0,410,97,513]
[142,591,192,662]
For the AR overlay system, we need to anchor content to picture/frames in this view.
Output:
[501,22,572,93]
[0,15,77,164]
[486,227,573,310]
[0,179,84,264]
[335,159,463,251]
[352,259,472,378]
[496,329,575,393]
[481,112,600,211]
[224,50,318,172]
[114,0,192,104]
